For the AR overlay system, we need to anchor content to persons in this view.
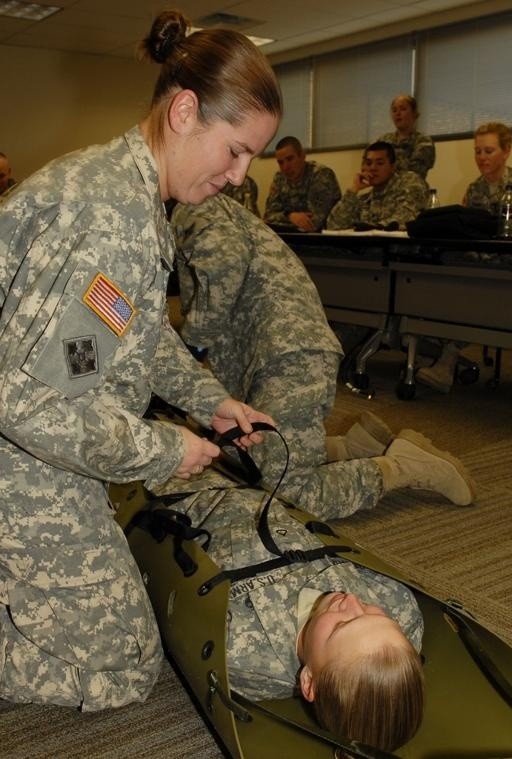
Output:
[151,467,426,759]
[262,135,342,233]
[168,193,477,523]
[0,9,285,714]
[414,121,512,394]
[0,152,22,195]
[360,94,437,181]
[219,175,260,218]
[325,140,429,232]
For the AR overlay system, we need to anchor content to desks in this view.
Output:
[277,232,511,400]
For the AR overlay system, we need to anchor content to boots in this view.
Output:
[369,429,478,508]
[415,338,461,395]
[324,409,396,464]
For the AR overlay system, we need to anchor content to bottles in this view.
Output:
[497,184,512,240]
[425,189,440,211]
[242,192,253,214]
